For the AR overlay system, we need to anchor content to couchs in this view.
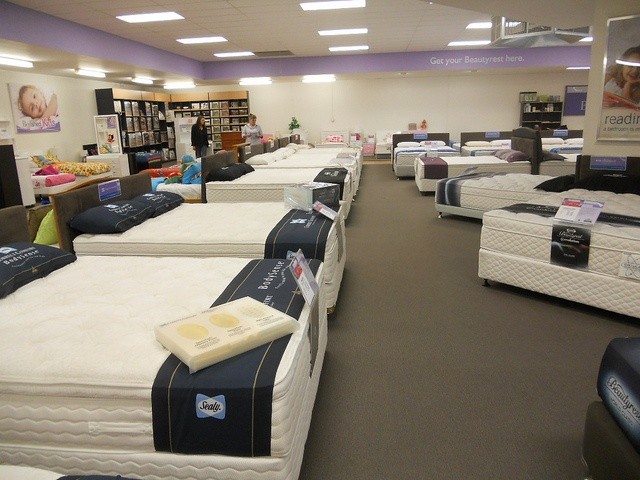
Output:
[23,146,112,197]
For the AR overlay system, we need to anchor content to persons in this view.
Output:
[241,114,264,145]
[17,85,58,120]
[603,46,640,103]
[191,116,209,158]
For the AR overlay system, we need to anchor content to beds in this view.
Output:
[0,204,328,480]
[392,133,456,180]
[582,338,640,479]
[376,130,401,159]
[202,150,350,203]
[316,129,349,148]
[512,127,577,175]
[449,140,460,149]
[265,139,361,195]
[138,155,201,199]
[479,155,639,320]
[45,170,346,308]
[279,137,364,169]
[414,136,537,195]
[240,143,351,219]
[434,155,582,221]
[536,130,583,154]
[460,130,512,155]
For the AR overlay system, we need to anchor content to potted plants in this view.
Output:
[289,116,301,134]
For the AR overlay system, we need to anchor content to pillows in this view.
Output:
[33,148,60,168]
[493,149,529,162]
[130,191,183,217]
[491,139,511,148]
[0,242,77,298]
[67,200,155,234]
[245,153,279,165]
[205,167,243,180]
[272,147,296,159]
[30,173,75,186]
[226,162,254,175]
[543,150,567,160]
[465,141,490,147]
[420,141,446,147]
[396,142,419,147]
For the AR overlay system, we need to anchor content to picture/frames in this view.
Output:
[595,14,639,142]
[8,82,61,132]
[93,114,123,154]
[563,86,586,116]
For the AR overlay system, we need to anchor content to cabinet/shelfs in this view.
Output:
[520,102,563,129]
[363,131,375,156]
[350,130,363,148]
[95,89,251,162]
[86,154,130,177]
[14,154,36,206]
